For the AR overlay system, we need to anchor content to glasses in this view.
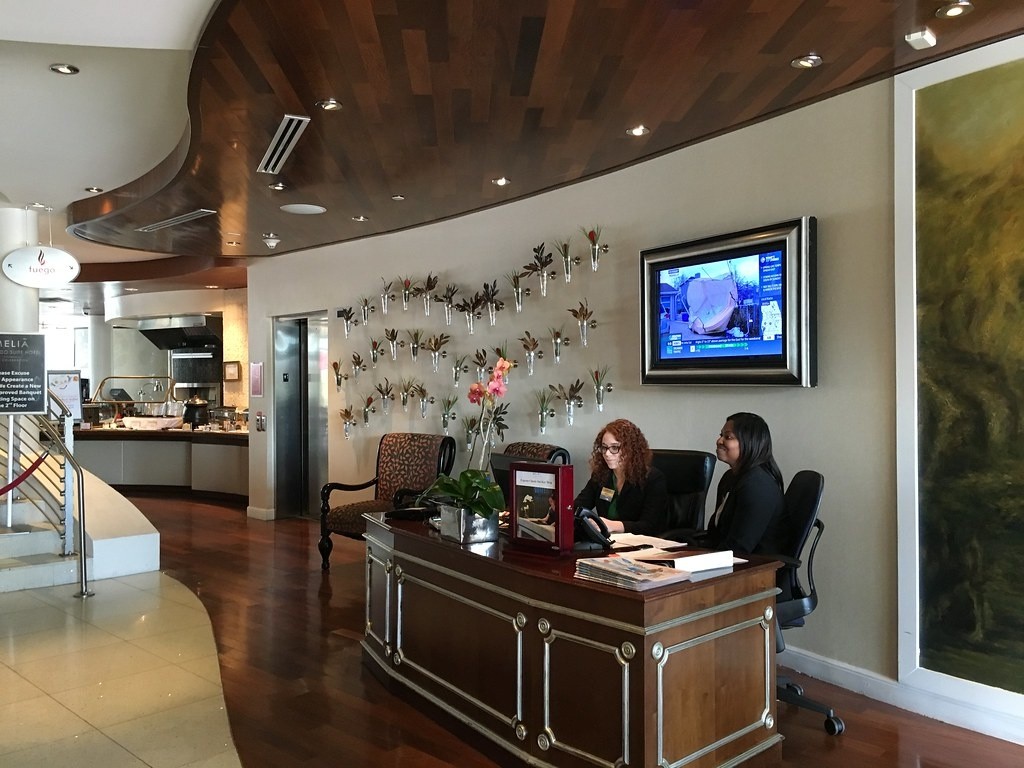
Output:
[597,445,621,454]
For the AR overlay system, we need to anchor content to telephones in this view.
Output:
[575,504,616,550]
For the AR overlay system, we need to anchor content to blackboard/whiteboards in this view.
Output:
[0,331,48,415]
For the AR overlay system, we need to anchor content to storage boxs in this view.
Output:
[83,406,99,425]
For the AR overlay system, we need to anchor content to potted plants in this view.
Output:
[332,236,609,452]
[415,468,501,544]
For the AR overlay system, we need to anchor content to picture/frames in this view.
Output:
[224,361,241,380]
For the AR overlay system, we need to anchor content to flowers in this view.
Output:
[396,274,418,289]
[587,361,610,385]
[360,393,381,408]
[468,359,511,405]
[329,358,343,374]
[367,336,383,348]
[576,224,603,244]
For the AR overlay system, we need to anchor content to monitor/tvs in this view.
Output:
[488,452,550,512]
[110,389,134,408]
[640,214,817,389]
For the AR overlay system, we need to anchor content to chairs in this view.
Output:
[318,433,455,573]
[502,442,569,465]
[775,469,845,735]
[646,449,717,530]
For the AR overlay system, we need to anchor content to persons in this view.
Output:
[528,492,555,525]
[701,412,794,603]
[574,419,685,537]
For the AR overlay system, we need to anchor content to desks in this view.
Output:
[359,513,784,768]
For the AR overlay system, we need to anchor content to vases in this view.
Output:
[594,385,604,412]
[362,408,371,429]
[334,374,342,392]
[589,244,600,272]
[369,348,378,369]
[401,290,409,310]
